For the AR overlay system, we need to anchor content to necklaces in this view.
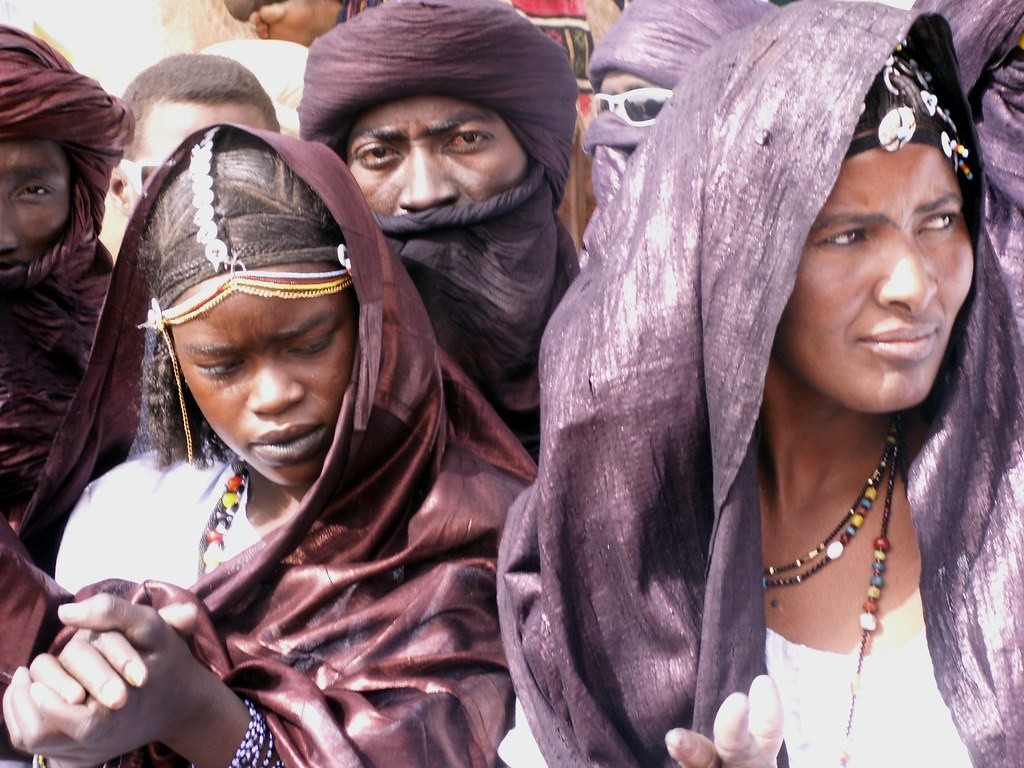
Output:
[760,422,901,767]
[205,458,248,572]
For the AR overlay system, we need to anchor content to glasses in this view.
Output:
[591,86,674,127]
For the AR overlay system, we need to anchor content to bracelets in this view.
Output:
[31,753,122,768]
[229,700,287,768]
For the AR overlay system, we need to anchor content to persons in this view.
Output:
[498,0,1023,768]
[912,1,1023,315]
[0,122,537,768]
[0,0,780,533]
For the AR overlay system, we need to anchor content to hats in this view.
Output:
[296,1,578,214]
